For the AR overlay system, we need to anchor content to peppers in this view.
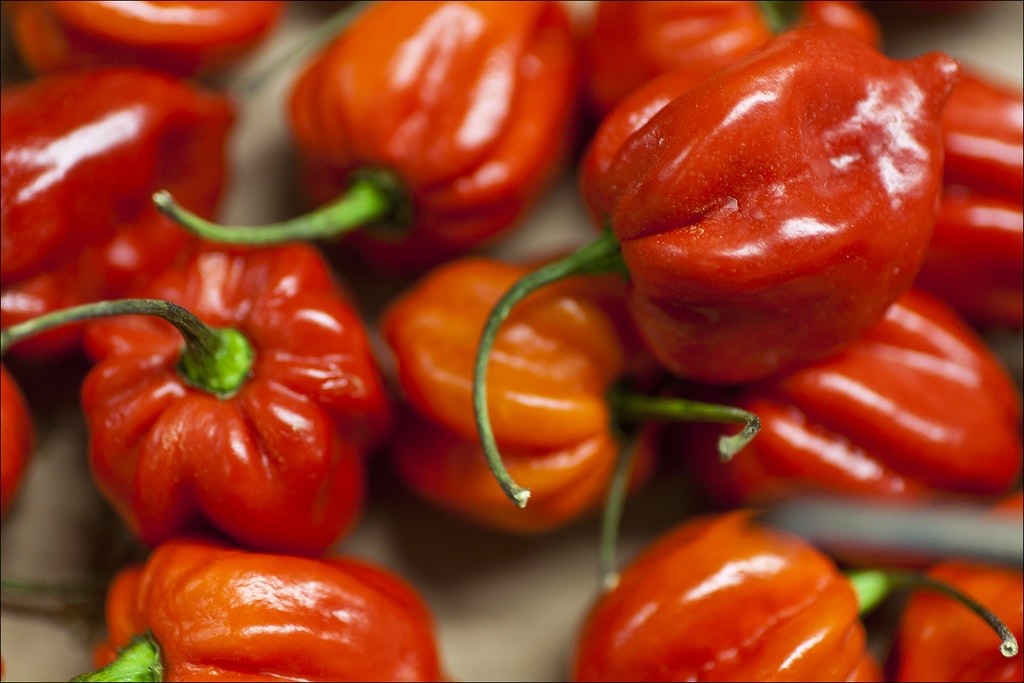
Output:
[0,0,1024,683]
